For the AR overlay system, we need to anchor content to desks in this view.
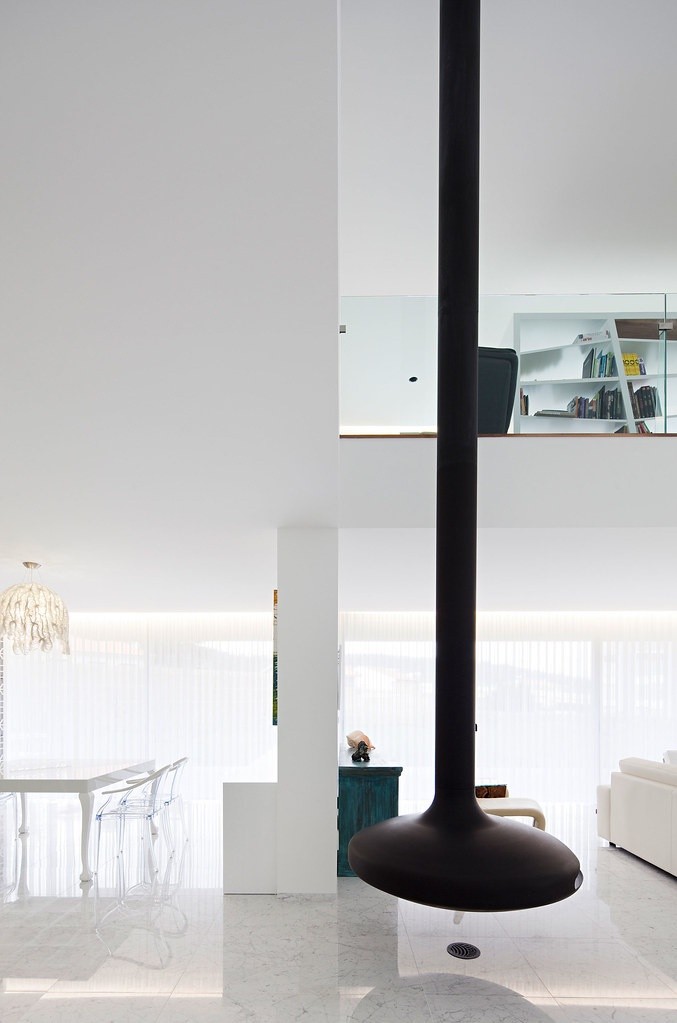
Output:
[475,796,546,831]
[0,757,157,882]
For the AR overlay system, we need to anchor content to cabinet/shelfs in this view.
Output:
[223,762,278,895]
[521,319,677,433]
[337,743,404,878]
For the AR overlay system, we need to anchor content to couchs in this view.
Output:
[595,748,677,878]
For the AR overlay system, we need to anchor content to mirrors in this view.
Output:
[339,292,677,439]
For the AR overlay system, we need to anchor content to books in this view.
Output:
[635,421,649,433]
[582,348,617,378]
[627,381,663,418]
[621,352,647,376]
[519,385,622,419]
[613,424,626,433]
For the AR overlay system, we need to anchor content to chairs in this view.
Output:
[120,755,191,856]
[94,764,172,875]
